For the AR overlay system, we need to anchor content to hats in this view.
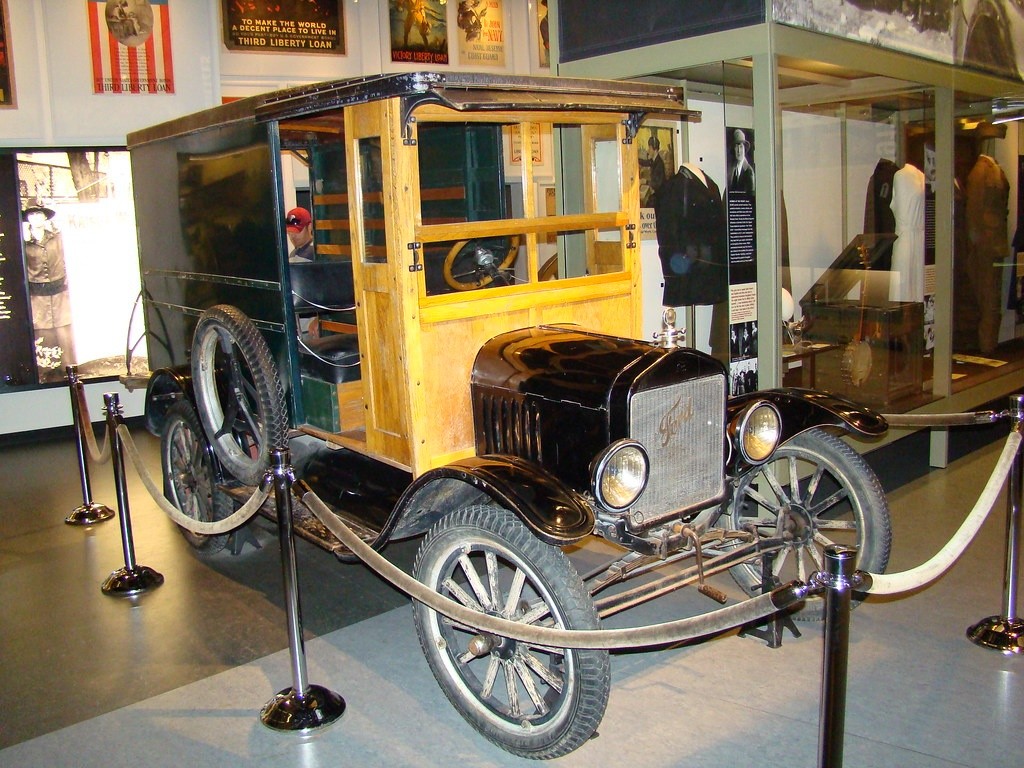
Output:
[286,207,311,234]
[21,199,55,222]
[729,129,750,161]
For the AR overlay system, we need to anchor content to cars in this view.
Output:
[119,70,894,762]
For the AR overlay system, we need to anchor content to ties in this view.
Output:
[736,167,739,182]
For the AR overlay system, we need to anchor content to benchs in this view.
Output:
[289,248,498,434]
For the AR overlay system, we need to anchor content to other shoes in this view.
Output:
[38,366,58,384]
[63,366,78,378]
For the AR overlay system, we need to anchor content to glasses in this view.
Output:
[284,218,309,226]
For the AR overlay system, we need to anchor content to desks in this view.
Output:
[781,340,842,390]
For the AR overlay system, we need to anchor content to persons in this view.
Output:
[638,136,668,208]
[286,207,317,262]
[731,322,757,361]
[735,368,758,395]
[722,129,756,214]
[21,199,78,374]
[655,164,729,308]
[964,155,1011,358]
